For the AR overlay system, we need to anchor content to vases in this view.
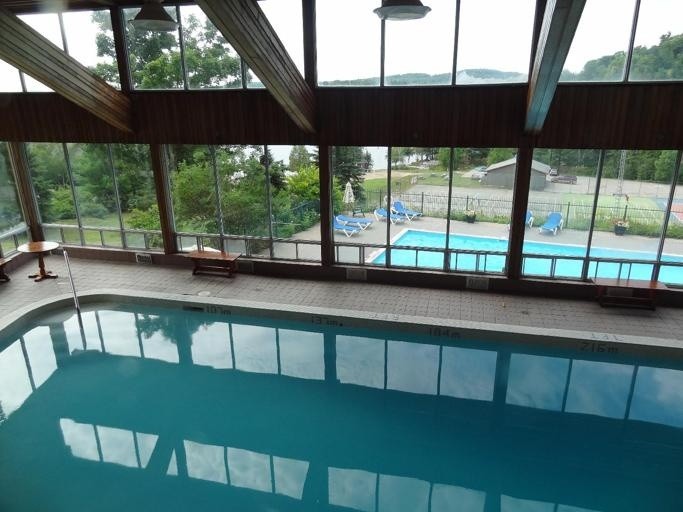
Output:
[466,215,475,223]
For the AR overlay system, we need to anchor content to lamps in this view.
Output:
[126,0,178,35]
[370,0,431,23]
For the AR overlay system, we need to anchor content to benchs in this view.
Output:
[0,250,22,284]
[588,275,673,311]
[182,249,242,279]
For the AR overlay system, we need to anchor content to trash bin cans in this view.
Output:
[466,215,476,224]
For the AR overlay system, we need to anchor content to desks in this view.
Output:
[16,241,60,283]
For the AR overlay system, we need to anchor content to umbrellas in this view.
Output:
[339,181,356,205]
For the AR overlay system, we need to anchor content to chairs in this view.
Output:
[527,210,565,236]
[332,214,373,238]
[372,200,423,226]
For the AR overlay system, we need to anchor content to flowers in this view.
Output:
[612,217,629,228]
[464,210,478,217]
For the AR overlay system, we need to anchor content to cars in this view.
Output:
[551,175,578,185]
[549,169,559,176]
